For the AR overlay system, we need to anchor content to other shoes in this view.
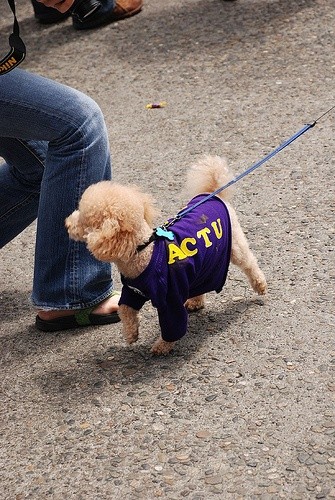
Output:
[113,0,144,20]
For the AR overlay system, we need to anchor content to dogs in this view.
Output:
[64,152,268,359]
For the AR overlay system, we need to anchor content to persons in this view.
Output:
[31,0,144,30]
[0,67,121,333]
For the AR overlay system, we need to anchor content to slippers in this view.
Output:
[35,291,121,332]
